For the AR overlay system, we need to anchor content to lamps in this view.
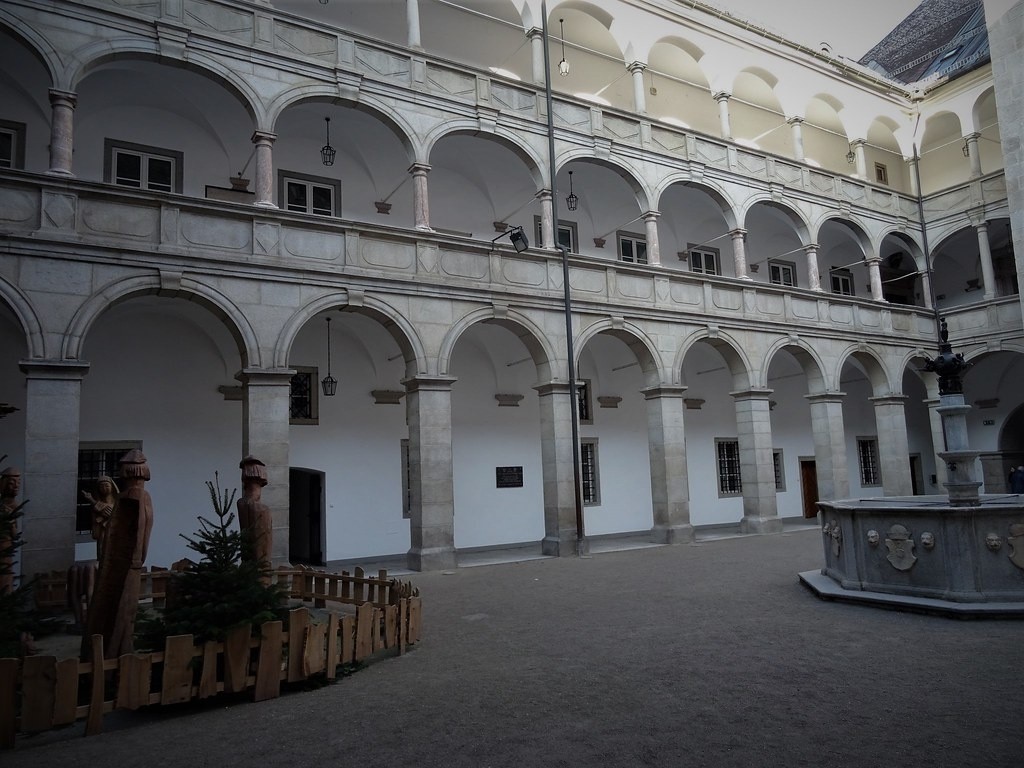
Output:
[845,143,856,164]
[962,142,970,157]
[489,226,530,254]
[321,317,338,397]
[558,19,570,78]
[566,171,578,211]
[320,117,337,167]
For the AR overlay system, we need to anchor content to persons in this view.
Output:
[82,476,120,561]
[81,448,154,654]
[237,455,273,563]
[0,466,21,596]
